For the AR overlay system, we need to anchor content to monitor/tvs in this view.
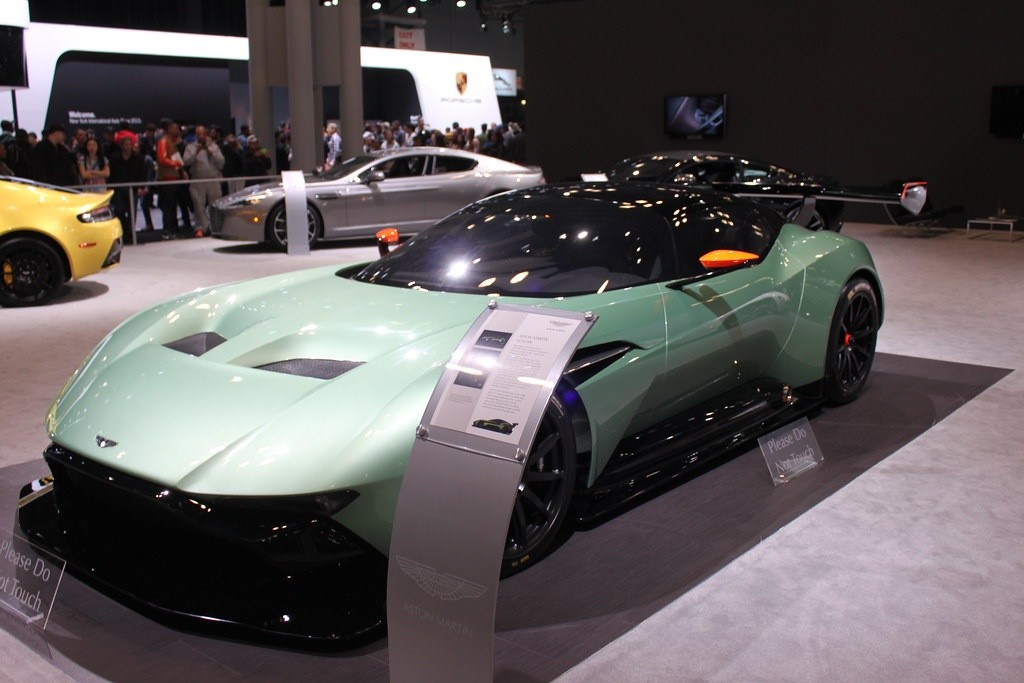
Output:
[664,93,727,141]
[990,84,1024,138]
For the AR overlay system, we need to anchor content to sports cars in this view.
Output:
[16,177,885,655]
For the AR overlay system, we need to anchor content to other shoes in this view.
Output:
[161,234,170,239]
[195,230,203,237]
[142,226,154,231]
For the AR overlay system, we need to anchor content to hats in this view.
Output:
[247,134,258,142]
[362,131,372,138]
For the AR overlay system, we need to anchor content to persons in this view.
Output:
[0,114,528,239]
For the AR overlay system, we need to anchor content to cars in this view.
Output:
[574,149,848,234]
[0,175,125,309]
[206,146,548,252]
